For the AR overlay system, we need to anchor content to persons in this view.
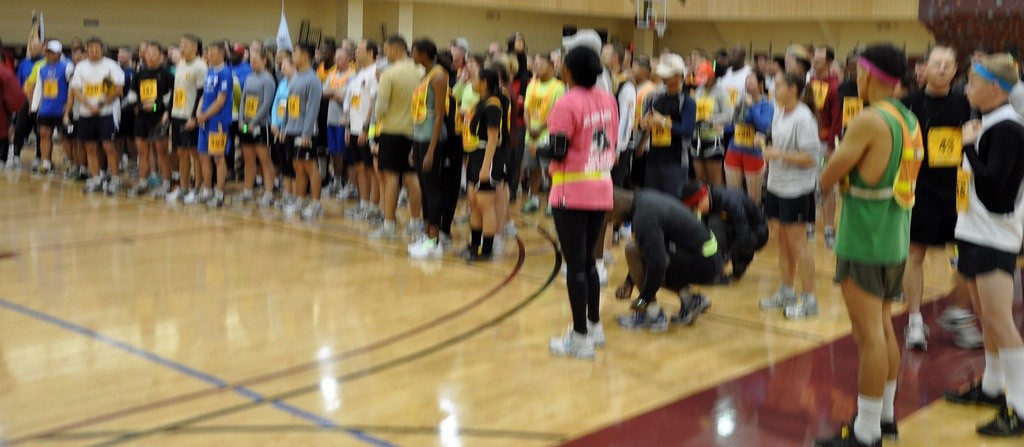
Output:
[944,54,1024,439]
[0,35,529,263]
[758,71,823,320]
[901,46,986,352]
[603,185,723,333]
[526,28,769,296]
[530,44,620,359]
[758,43,1023,234]
[813,43,925,447]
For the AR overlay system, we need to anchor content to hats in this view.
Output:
[656,53,684,79]
[562,27,602,53]
[46,39,63,54]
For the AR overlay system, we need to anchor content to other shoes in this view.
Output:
[806,223,814,239]
[759,290,798,311]
[7,156,516,261]
[562,260,609,284]
[824,228,836,250]
[670,291,712,326]
[549,328,594,358]
[522,198,541,214]
[617,306,667,332]
[545,201,552,216]
[783,296,818,319]
[586,325,604,347]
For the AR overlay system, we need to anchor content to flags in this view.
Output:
[27,11,46,56]
[276,17,293,51]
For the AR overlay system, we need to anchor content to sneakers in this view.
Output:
[812,422,883,447]
[906,320,930,351]
[853,415,898,437]
[936,308,986,349]
[946,375,1006,409]
[976,404,1024,436]
